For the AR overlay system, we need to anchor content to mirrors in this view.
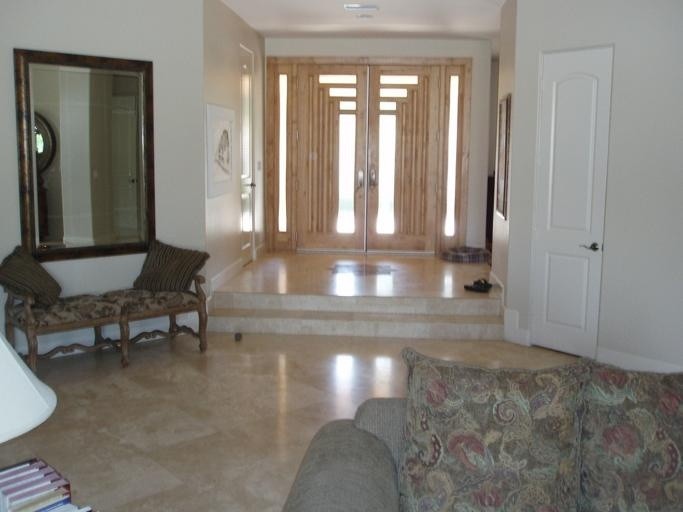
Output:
[13,48,156,264]
[35,112,56,173]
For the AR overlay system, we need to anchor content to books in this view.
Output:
[0,457,97,512]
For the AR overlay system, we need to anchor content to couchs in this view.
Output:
[281,399,410,510]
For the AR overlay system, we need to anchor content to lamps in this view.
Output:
[0,333,57,444]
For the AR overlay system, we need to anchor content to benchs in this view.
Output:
[3,275,208,377]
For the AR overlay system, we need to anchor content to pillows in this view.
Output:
[580,361,683,512]
[0,244,62,308]
[134,239,209,292]
[401,347,597,512]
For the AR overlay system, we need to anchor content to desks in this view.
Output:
[0,457,98,512]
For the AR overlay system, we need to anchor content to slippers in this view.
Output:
[464,279,494,293]
